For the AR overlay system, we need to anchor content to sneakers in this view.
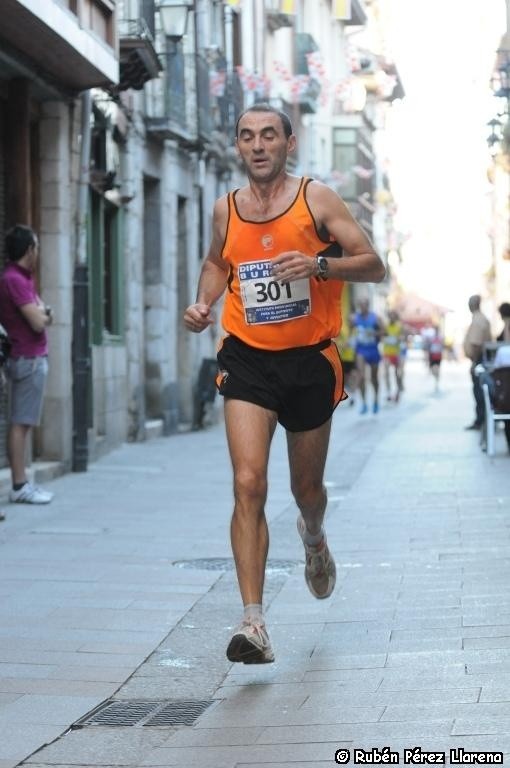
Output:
[298,514,336,599]
[227,618,274,664]
[9,480,53,504]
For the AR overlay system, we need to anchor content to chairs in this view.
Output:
[481,384,510,457]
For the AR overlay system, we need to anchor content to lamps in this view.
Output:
[156,0,194,56]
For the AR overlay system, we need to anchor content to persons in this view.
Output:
[184,103,386,665]
[337,295,510,452]
[0,223,54,504]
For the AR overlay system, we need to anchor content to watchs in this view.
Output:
[316,255,328,282]
